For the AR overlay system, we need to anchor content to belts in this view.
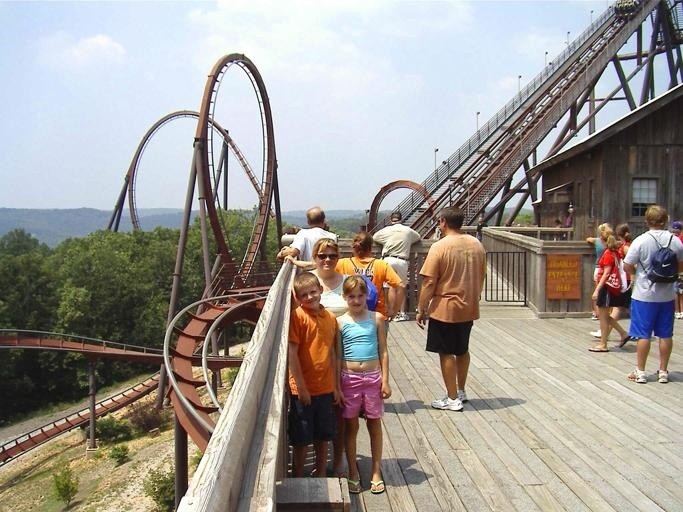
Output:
[381,254,408,261]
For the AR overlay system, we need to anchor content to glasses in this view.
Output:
[316,253,339,260]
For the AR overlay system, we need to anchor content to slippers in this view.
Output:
[618,336,632,348]
[588,347,610,352]
[370,480,385,494]
[347,477,361,493]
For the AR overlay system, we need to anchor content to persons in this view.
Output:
[333,275,391,494]
[623,205,683,383]
[436,220,445,240]
[476,215,486,241]
[276,208,420,418]
[288,272,339,478]
[565,213,572,228]
[415,207,486,410]
[554,220,566,240]
[587,223,639,352]
[671,222,683,319]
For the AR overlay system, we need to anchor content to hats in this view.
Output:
[391,212,401,222]
[672,222,683,230]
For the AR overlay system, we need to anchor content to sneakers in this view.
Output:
[674,311,683,319]
[400,312,410,321]
[446,389,467,402]
[590,329,602,337]
[627,369,649,383]
[392,312,401,321]
[656,369,669,383]
[431,397,464,411]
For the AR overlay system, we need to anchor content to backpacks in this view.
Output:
[638,232,679,284]
[350,256,378,311]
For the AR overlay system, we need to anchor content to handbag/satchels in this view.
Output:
[597,265,622,297]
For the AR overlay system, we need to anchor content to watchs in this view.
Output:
[415,308,424,314]
